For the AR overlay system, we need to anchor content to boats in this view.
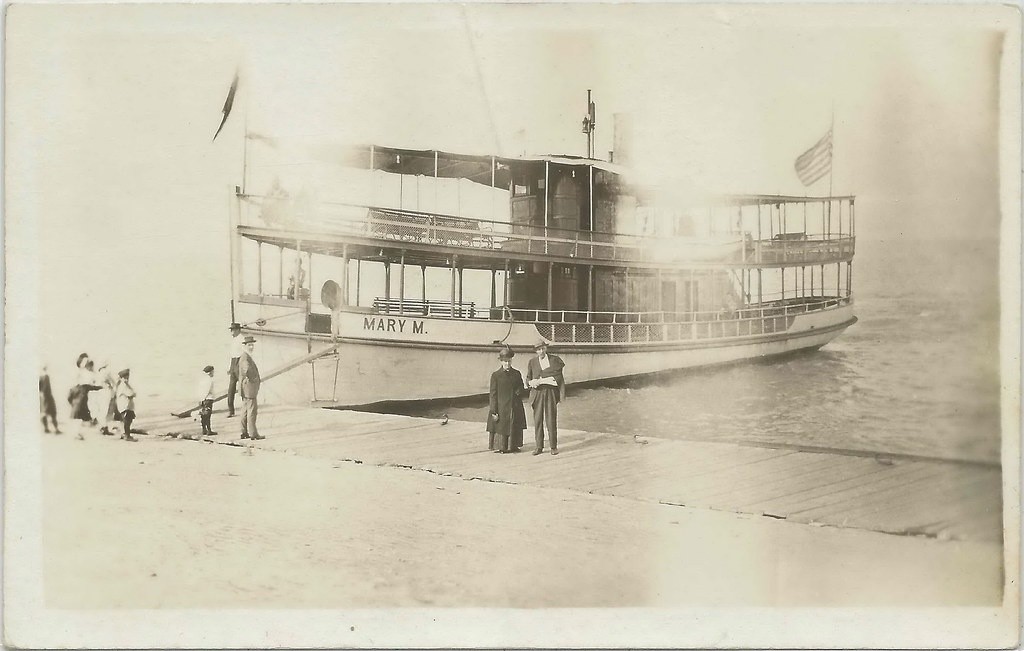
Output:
[231,89,860,411]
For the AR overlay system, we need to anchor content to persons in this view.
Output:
[484,349,528,452]
[287,256,307,299]
[66,353,139,439]
[524,341,567,455]
[225,321,249,418]
[198,364,218,435]
[238,335,266,440]
[38,365,61,436]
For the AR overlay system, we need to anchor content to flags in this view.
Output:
[793,127,837,189]
[209,57,240,136]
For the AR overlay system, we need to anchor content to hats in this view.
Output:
[203,366,214,373]
[117,369,129,377]
[228,323,243,329]
[497,349,514,360]
[534,339,547,350]
[242,336,257,344]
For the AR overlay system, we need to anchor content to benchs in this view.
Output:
[363,207,808,321]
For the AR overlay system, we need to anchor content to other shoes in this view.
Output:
[121,435,140,442]
[45,429,62,434]
[227,414,236,418]
[499,446,521,455]
[100,427,115,435]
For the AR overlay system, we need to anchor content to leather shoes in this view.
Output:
[202,430,217,435]
[532,447,559,455]
[241,433,266,440]
